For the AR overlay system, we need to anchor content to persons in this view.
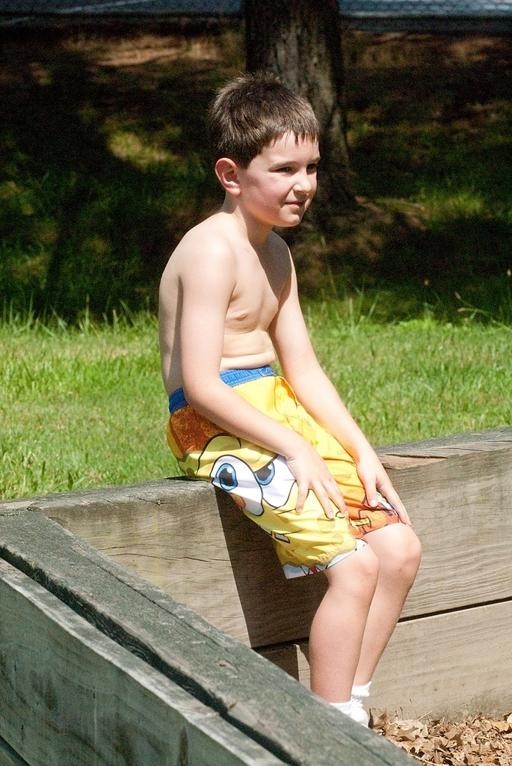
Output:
[158,77,421,730]
